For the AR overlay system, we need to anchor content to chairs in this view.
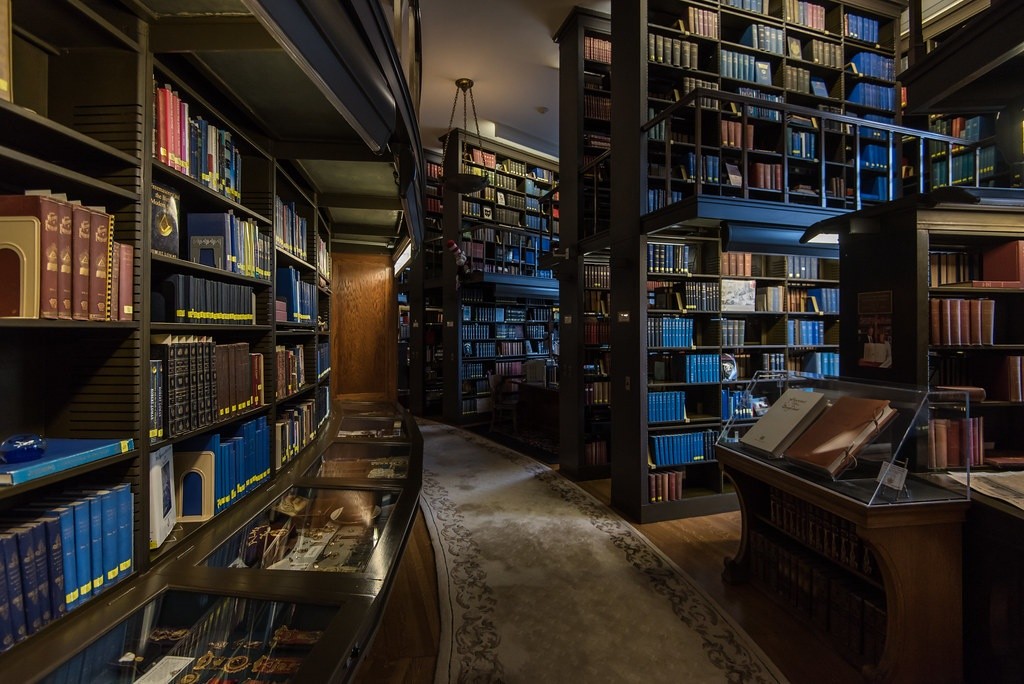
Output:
[487,369,521,434]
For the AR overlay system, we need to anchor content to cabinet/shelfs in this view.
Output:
[1,0,1024,684]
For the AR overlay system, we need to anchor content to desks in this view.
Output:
[509,378,559,447]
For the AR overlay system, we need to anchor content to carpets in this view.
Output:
[489,421,560,453]
[412,415,794,684]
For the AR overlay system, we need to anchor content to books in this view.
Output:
[400,148,559,416]
[0,89,330,655]
[584,0,895,505]
[36,486,264,684]
[930,117,997,190]
[739,252,1024,483]
[749,486,886,660]
